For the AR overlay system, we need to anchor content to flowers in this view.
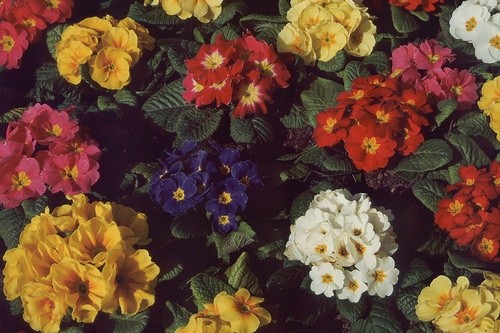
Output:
[0,0,500,333]
